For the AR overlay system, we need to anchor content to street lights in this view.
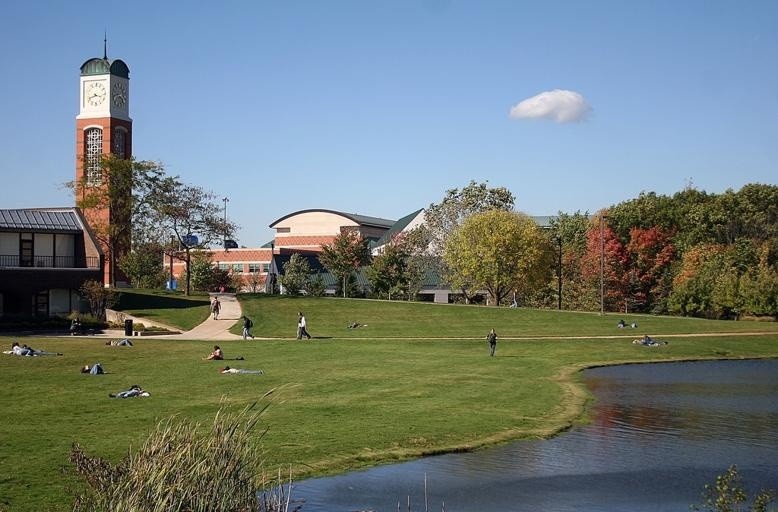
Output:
[597,214,609,312]
[551,234,564,312]
[220,196,230,248]
[269,242,275,295]
[168,232,175,290]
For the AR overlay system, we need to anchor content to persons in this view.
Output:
[219,285,225,296]
[617,320,625,328]
[221,365,264,374]
[82,362,104,375]
[509,288,519,309]
[242,315,254,339]
[296,311,311,340]
[210,296,221,320]
[105,338,133,347]
[485,327,499,356]
[11,342,64,358]
[347,322,361,329]
[109,388,148,399]
[632,335,668,347]
[202,345,223,360]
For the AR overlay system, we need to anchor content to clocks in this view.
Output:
[83,79,107,108]
[110,78,128,109]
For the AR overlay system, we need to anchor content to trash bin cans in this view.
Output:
[125,319,133,336]
